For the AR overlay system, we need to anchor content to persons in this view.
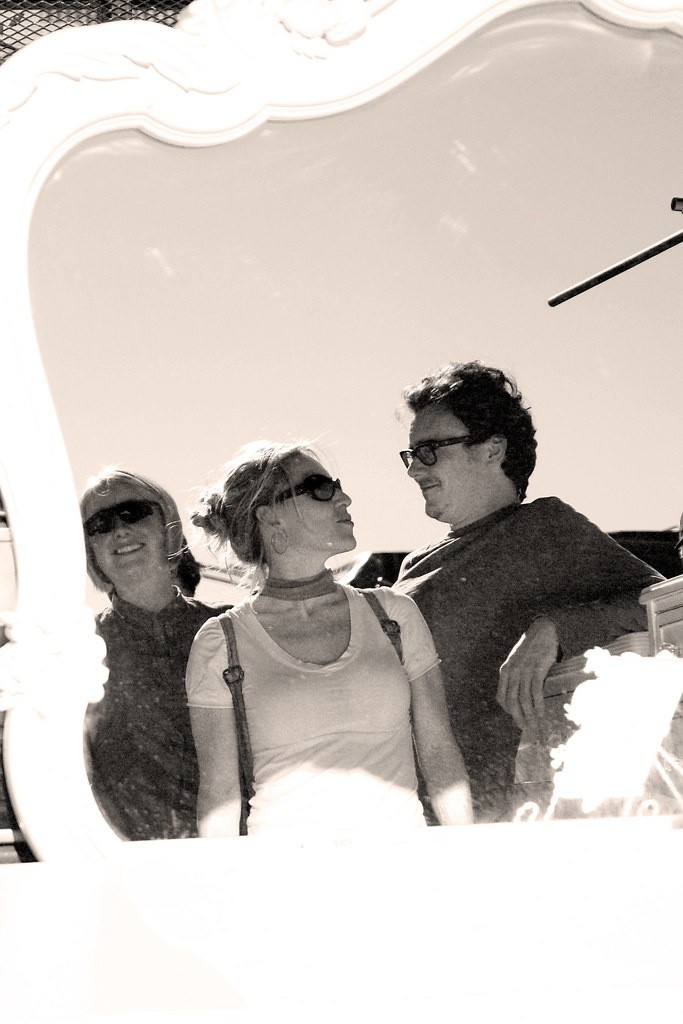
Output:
[81,469,236,843]
[185,447,476,839]
[388,356,667,826]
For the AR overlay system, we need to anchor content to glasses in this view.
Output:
[399,435,475,468]
[252,474,344,509]
[82,498,157,536]
